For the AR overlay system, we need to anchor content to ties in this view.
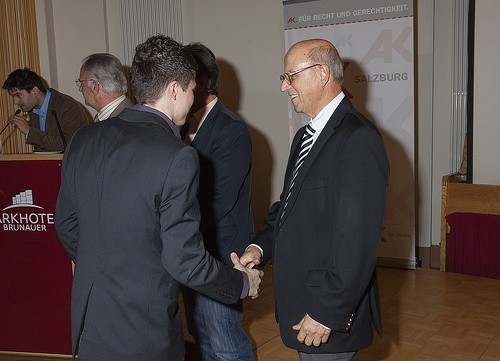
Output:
[278,123,315,225]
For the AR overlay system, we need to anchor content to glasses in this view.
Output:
[279,64,322,85]
[75,79,100,87]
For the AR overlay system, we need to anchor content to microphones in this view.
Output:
[0,109,21,135]
[51,110,67,154]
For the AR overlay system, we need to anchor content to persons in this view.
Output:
[2,67,93,152]
[75,53,132,122]
[180,41,256,361]
[55,32,261,361]
[240,39,390,361]
